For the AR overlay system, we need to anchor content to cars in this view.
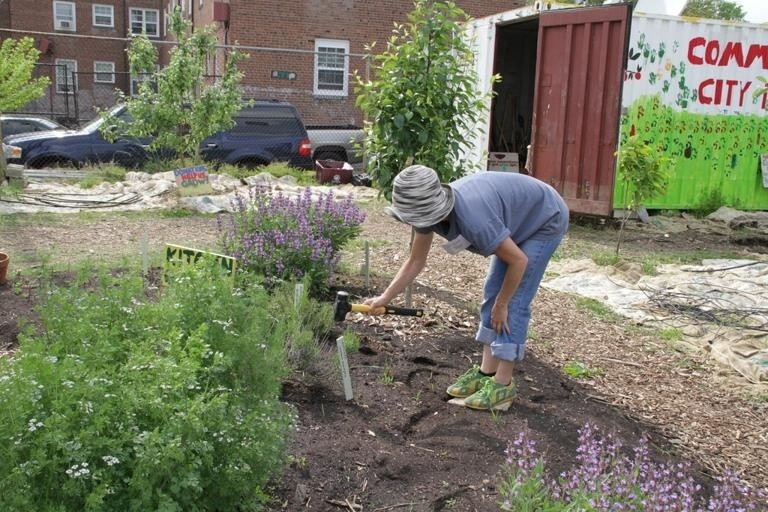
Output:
[0,101,71,139]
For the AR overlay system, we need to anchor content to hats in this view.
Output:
[382,164,457,230]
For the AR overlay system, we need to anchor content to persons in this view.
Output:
[360,164,570,411]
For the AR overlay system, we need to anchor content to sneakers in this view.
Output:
[445,365,518,412]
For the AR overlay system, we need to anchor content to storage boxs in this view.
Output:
[315,160,353,185]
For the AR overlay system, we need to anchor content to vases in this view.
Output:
[0,251,9,284]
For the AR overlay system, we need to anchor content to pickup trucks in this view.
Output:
[304,124,364,165]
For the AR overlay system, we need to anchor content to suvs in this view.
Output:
[2,98,314,173]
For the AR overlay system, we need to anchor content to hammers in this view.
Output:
[334,291,423,321]
